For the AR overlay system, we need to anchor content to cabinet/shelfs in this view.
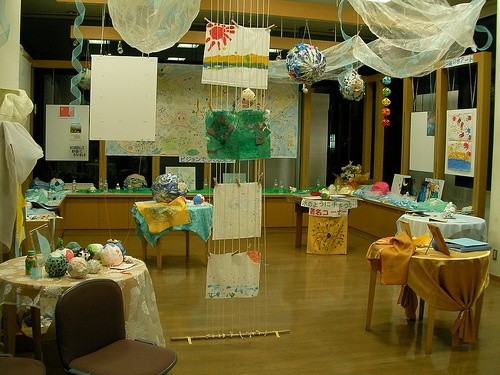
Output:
[63,189,338,231]
[20,185,64,254]
[348,187,405,239]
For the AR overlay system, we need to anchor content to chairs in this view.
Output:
[28,223,55,254]
[0,356,46,375]
[55,279,177,375]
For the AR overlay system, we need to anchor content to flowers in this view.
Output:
[371,181,390,195]
[340,161,375,181]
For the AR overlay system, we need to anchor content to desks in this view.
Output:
[131,200,213,271]
[363,234,494,355]
[394,212,487,242]
[0,251,169,375]
[286,193,359,247]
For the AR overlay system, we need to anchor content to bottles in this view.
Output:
[25,249,37,276]
[128,180,133,192]
[279,181,283,194]
[116,183,120,194]
[315,177,321,187]
[72,180,76,193]
[103,180,108,193]
[99,177,103,189]
[274,179,278,190]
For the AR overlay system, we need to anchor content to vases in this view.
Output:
[347,180,361,188]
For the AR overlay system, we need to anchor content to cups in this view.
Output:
[30,268,42,278]
